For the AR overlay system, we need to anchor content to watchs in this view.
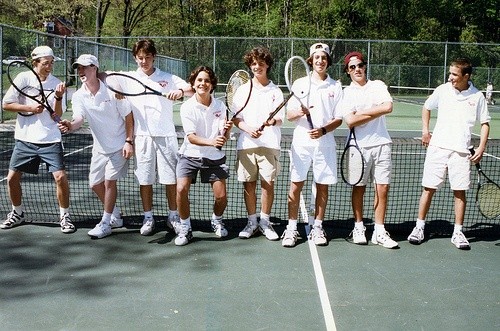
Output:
[322,127,326,135]
[125,140,134,145]
[54,96,62,101]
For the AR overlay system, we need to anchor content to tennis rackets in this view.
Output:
[216,69,252,150]
[17,82,71,116]
[285,56,314,130]
[7,61,69,130]
[251,92,294,138]
[341,112,364,186]
[468,146,500,220]
[104,73,183,102]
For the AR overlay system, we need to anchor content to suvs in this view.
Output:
[2,56,27,67]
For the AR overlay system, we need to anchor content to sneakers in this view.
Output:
[87,222,112,240]
[451,231,470,249]
[96,214,123,230]
[371,230,399,249]
[311,226,327,246]
[407,226,424,244]
[166,215,181,235]
[258,219,280,241]
[211,218,229,239]
[139,219,155,236]
[0,210,25,229]
[281,228,300,248]
[238,221,259,239]
[59,212,75,233]
[175,225,193,245]
[353,226,367,245]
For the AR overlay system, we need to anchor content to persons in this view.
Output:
[175,66,233,245]
[486,82,494,105]
[232,48,285,241]
[56,54,134,238]
[282,43,344,247]
[0,45,75,233]
[342,53,399,249]
[407,59,491,249]
[98,39,195,236]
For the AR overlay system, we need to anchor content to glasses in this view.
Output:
[347,61,368,72]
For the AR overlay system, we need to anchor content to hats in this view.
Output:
[342,51,364,73]
[306,43,331,66]
[72,54,99,69]
[31,45,59,62]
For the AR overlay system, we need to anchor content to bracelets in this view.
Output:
[273,119,276,125]
[178,89,184,96]
